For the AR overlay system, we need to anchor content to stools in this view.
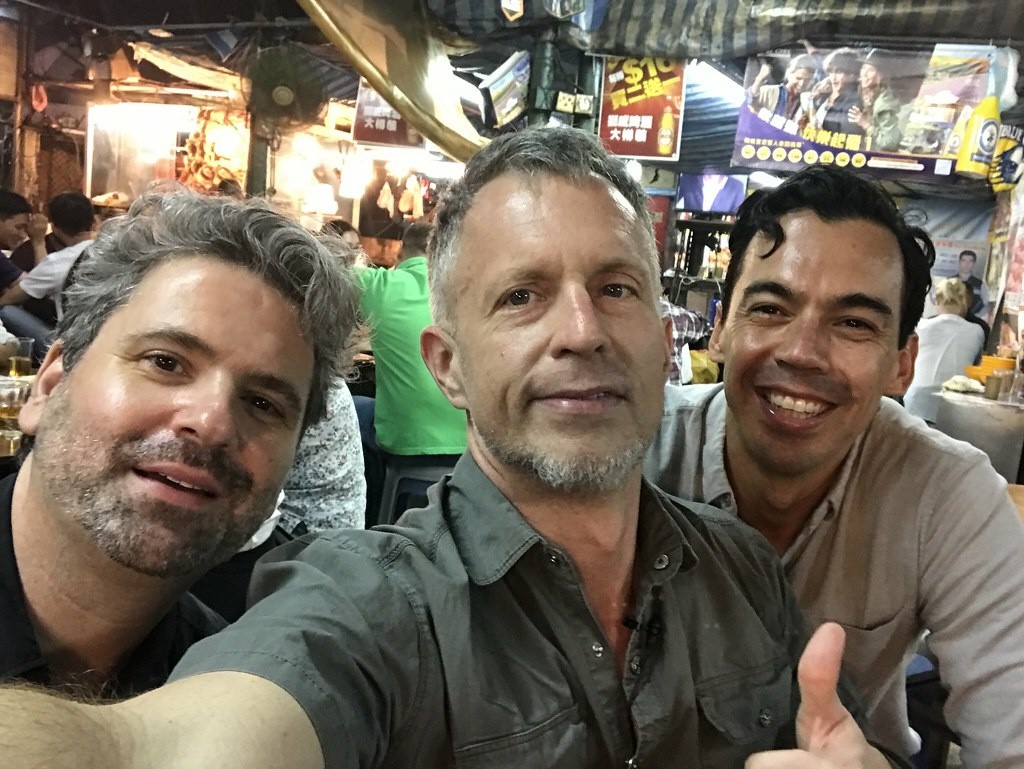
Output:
[376,468,456,528]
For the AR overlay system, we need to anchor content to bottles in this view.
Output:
[956,94,1000,179]
[709,292,720,327]
[657,106,674,155]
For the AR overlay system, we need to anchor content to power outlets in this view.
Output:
[555,89,577,113]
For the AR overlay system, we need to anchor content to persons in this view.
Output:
[747,37,903,152]
[947,250,989,318]
[2,124,919,769]
[640,161,1024,769]
[1,128,1024,575]
[1,185,360,704]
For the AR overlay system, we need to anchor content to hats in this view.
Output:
[789,54,818,75]
[855,48,893,70]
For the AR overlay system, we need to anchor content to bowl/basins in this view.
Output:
[965,366,984,384]
[982,355,1016,382]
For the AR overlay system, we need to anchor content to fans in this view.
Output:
[239,44,330,152]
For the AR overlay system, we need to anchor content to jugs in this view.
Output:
[56,111,86,128]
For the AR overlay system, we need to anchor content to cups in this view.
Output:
[6,336,34,378]
[1014,371,1024,405]
[994,370,1016,402]
[986,374,1002,400]
[0,429,24,456]
[0,380,28,418]
